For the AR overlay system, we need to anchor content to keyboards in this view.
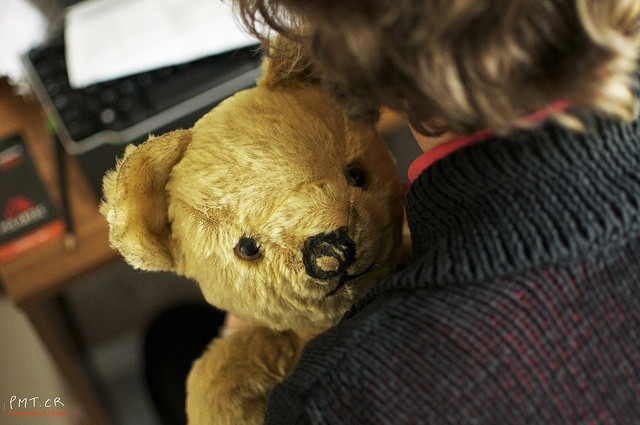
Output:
[18,35,262,158]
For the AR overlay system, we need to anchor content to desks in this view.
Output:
[1,66,400,425]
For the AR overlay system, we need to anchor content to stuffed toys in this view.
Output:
[98,26,413,425]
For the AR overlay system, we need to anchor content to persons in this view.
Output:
[224,0,640,424]
